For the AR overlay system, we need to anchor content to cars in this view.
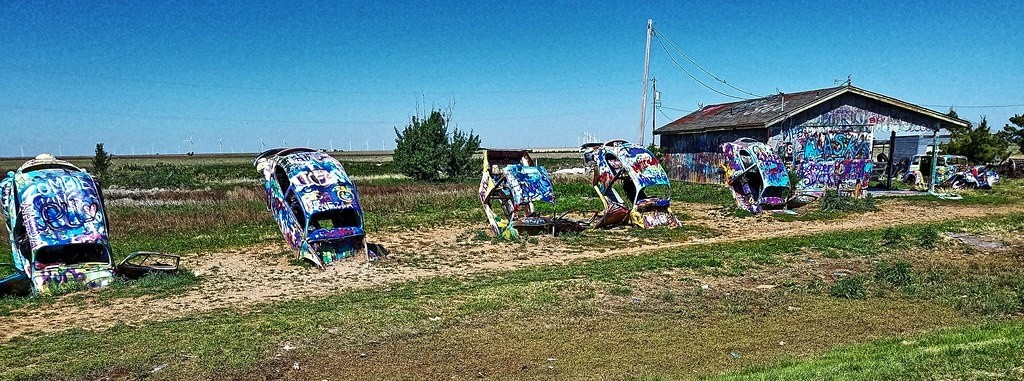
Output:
[579,138,682,231]
[0,153,180,303]
[478,148,599,241]
[721,135,818,217]
[252,147,389,269]
[942,162,999,191]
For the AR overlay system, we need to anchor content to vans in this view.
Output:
[903,155,968,192]
